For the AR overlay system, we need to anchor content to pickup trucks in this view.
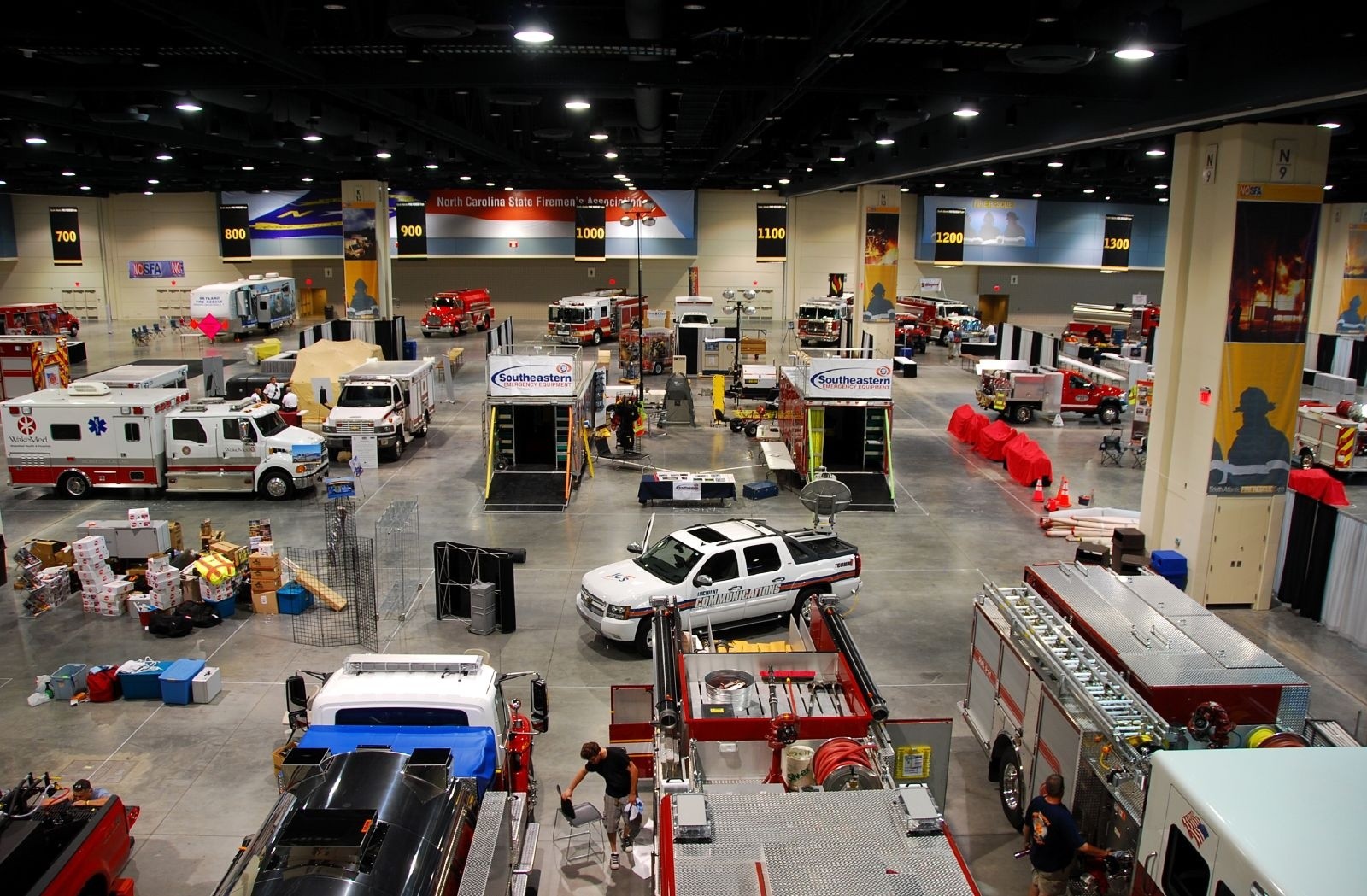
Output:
[575,511,864,659]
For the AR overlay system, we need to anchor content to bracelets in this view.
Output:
[85,800,89,806]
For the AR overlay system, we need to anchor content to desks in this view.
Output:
[436,347,464,383]
[959,354,979,372]
[638,474,737,505]
[180,333,204,349]
[598,350,611,384]
[759,441,796,480]
[756,425,781,458]
[894,356,917,377]
[1058,351,1154,387]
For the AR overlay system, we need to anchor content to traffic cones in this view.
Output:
[1053,474,1066,501]
[1032,474,1045,503]
[1054,480,1072,507]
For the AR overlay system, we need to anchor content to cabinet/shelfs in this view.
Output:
[703,337,738,373]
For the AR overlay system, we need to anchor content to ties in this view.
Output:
[273,383,278,398]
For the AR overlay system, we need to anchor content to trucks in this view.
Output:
[420,286,496,336]
[320,355,437,462]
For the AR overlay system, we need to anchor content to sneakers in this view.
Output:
[610,853,620,869]
[619,830,634,853]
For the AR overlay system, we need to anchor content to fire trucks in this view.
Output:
[0,334,71,403]
[606,593,982,896]
[796,292,853,348]
[957,557,1365,896]
[892,312,927,354]
[0,771,141,896]
[0,302,80,338]
[975,358,1130,424]
[895,294,984,348]
[1061,300,1161,347]
[547,287,652,346]
[208,648,550,896]
[1291,396,1367,485]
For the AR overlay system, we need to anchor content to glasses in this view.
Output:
[73,784,86,788]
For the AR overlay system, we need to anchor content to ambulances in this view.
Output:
[1,380,331,502]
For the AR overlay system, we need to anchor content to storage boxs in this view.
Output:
[1073,527,1187,591]
[899,347,912,359]
[51,658,222,703]
[26,506,347,618]
[326,476,355,498]
[249,337,282,364]
[743,480,779,500]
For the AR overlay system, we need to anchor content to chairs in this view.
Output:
[1099,435,1148,469]
[595,438,654,474]
[553,783,604,861]
[132,318,191,346]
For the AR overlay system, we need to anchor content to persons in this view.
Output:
[606,397,640,453]
[984,323,996,342]
[561,742,638,870]
[251,376,298,412]
[964,214,976,238]
[67,778,111,807]
[1005,212,1025,237]
[1337,296,1365,323]
[1021,775,1112,896]
[947,328,964,359]
[980,212,1001,240]
